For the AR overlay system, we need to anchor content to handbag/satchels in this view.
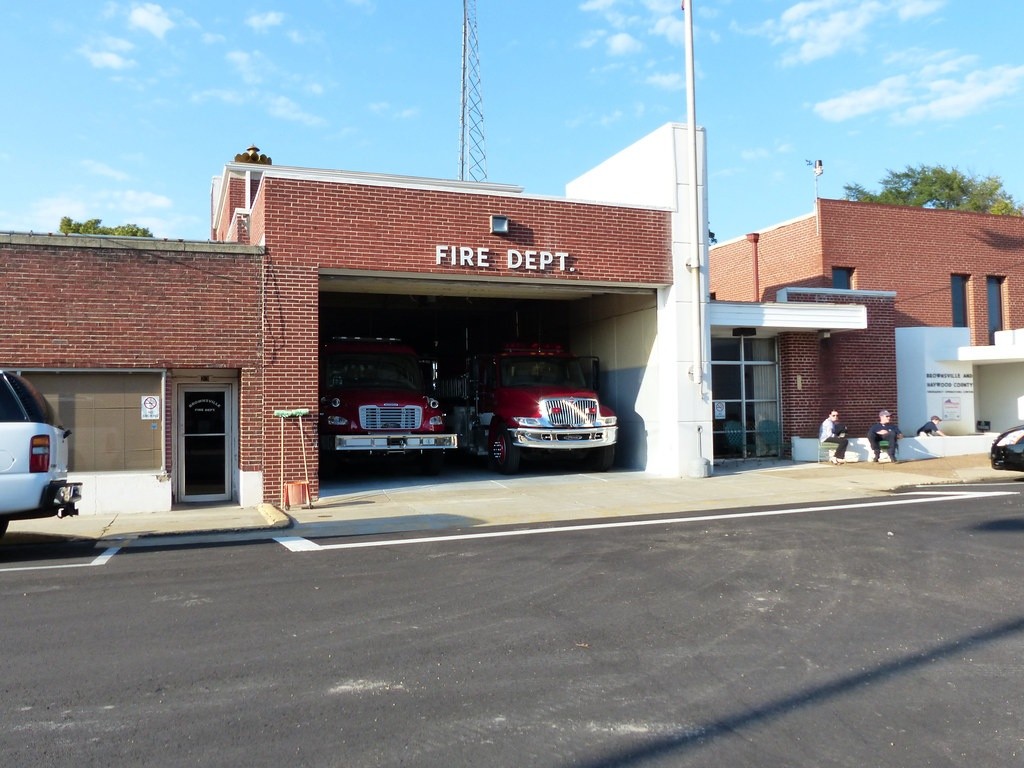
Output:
[878,450,891,463]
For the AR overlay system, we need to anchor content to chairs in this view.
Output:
[377,362,399,382]
[510,365,537,384]
[542,363,560,384]
[817,424,900,463]
[723,419,793,464]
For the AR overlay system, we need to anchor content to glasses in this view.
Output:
[882,415,890,417]
[831,414,838,417]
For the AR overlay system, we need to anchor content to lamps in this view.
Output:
[733,328,756,336]
[489,215,509,234]
[817,329,831,339]
[813,159,824,237]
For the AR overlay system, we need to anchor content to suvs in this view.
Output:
[0,367,84,540]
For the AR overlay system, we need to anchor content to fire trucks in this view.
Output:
[317,336,459,476]
[439,341,618,475]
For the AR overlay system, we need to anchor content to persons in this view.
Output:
[819,409,848,465]
[917,416,946,437]
[868,410,904,463]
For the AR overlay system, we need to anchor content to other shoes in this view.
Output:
[891,456,896,462]
[829,457,846,464]
[873,457,878,462]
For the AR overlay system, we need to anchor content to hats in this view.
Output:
[931,416,942,421]
[879,410,892,417]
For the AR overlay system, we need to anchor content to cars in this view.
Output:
[989,424,1024,473]
